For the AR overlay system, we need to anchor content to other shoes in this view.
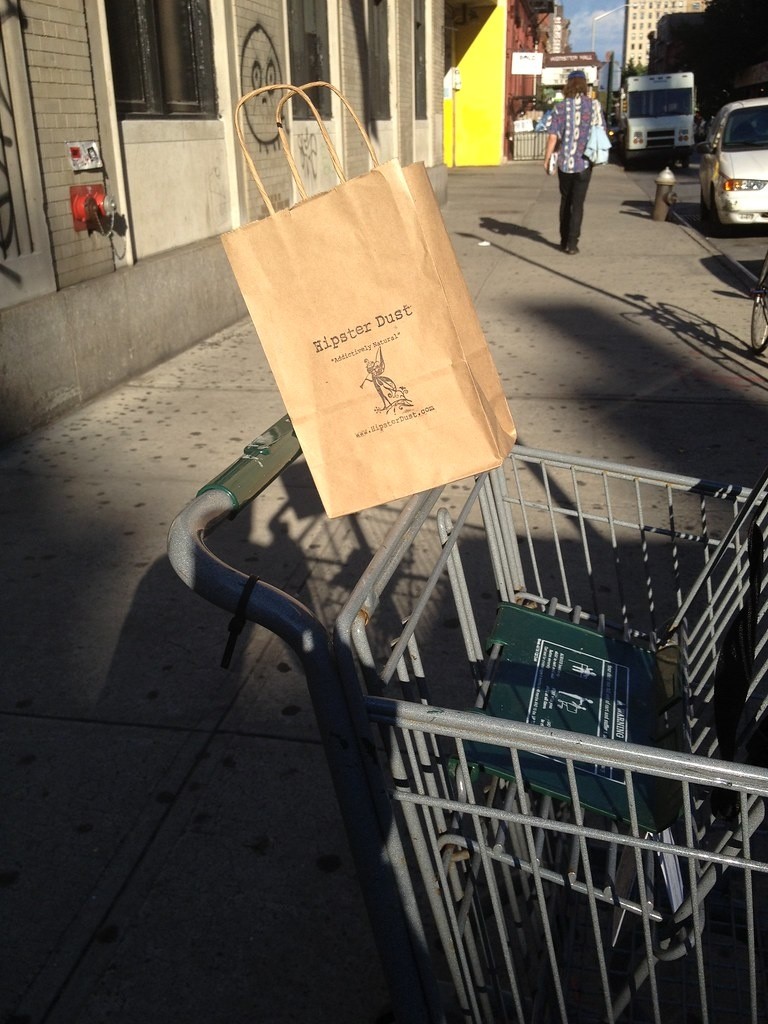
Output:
[559,237,579,255]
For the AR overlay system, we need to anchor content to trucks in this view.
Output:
[621,72,696,169]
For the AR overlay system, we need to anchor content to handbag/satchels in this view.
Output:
[583,100,612,166]
[221,81,516,520]
[548,153,557,176]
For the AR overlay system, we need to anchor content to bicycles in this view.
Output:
[748,250,768,354]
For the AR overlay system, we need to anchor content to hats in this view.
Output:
[567,71,585,78]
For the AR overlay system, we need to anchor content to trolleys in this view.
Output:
[165,411,768,1024]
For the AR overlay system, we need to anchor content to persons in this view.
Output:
[542,69,606,254]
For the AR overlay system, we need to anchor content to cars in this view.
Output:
[694,97,768,233]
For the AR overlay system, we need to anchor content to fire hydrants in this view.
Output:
[651,166,677,223]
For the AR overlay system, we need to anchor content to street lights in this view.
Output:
[591,3,637,53]
